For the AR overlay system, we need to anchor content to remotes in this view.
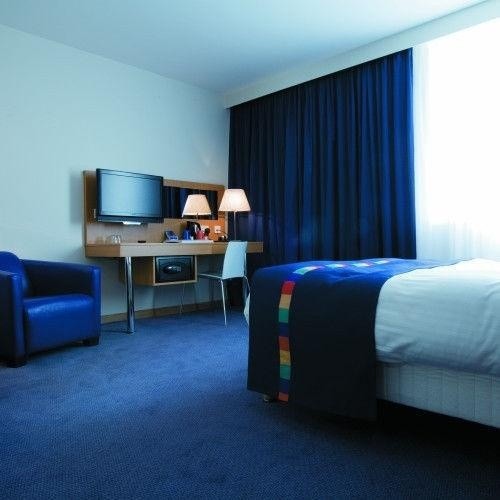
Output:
[138,240,146,243]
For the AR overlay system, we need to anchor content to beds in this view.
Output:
[251,254,500,433]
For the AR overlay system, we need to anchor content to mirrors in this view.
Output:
[163,187,217,220]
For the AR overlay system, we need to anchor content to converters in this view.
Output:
[205,228,210,236]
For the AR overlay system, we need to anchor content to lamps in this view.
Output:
[219,189,251,241]
[183,194,212,220]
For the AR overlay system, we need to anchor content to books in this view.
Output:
[163,239,216,244]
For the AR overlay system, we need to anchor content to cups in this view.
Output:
[110,234,122,242]
[138,231,147,243]
[182,229,190,240]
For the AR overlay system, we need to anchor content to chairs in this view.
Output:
[179,239,251,326]
[0,252,101,368]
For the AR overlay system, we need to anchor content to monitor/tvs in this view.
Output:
[96,169,164,225]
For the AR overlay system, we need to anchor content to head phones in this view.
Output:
[217,230,232,242]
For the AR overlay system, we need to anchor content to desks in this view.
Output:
[85,241,264,333]
